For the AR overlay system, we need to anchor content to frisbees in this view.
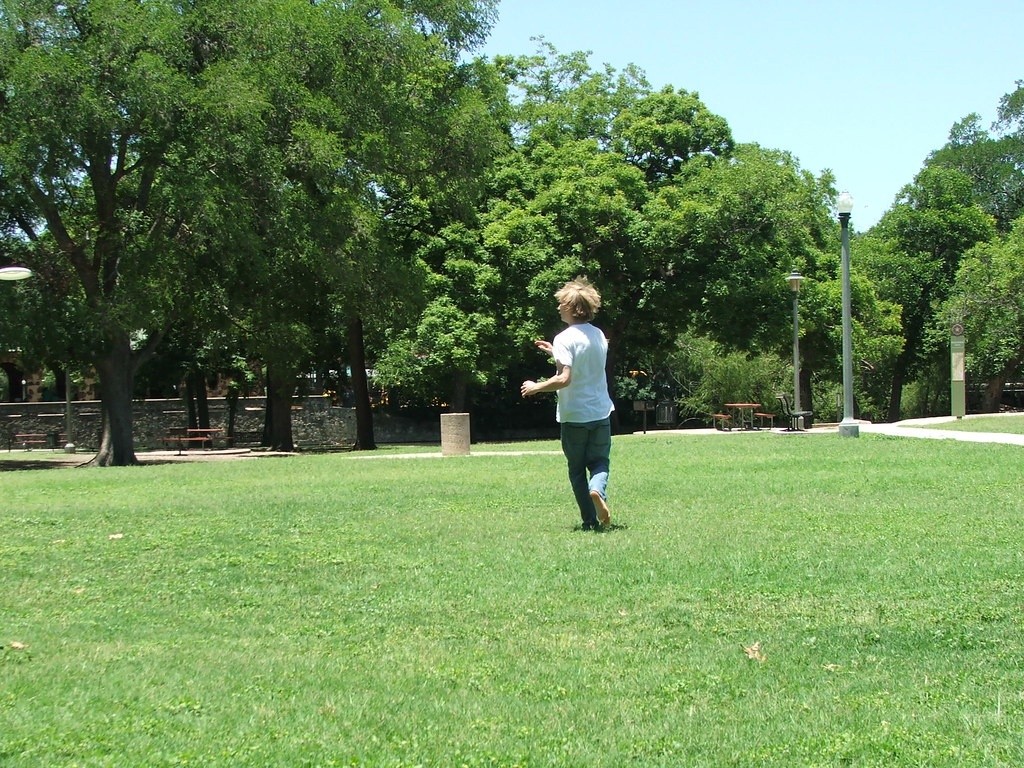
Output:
[0,267,33,281]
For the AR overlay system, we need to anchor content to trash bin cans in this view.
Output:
[441,413,470,456]
[656,402,677,426]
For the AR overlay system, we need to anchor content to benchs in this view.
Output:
[156,437,214,452]
[15,440,47,448]
[754,413,776,430]
[711,414,732,431]
[211,436,235,450]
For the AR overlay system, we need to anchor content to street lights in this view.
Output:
[835,188,860,438]
[785,272,806,432]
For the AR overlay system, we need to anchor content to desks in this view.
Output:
[14,433,49,449]
[724,403,762,431]
[165,429,223,451]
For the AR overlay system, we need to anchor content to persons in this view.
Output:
[521,280,615,529]
